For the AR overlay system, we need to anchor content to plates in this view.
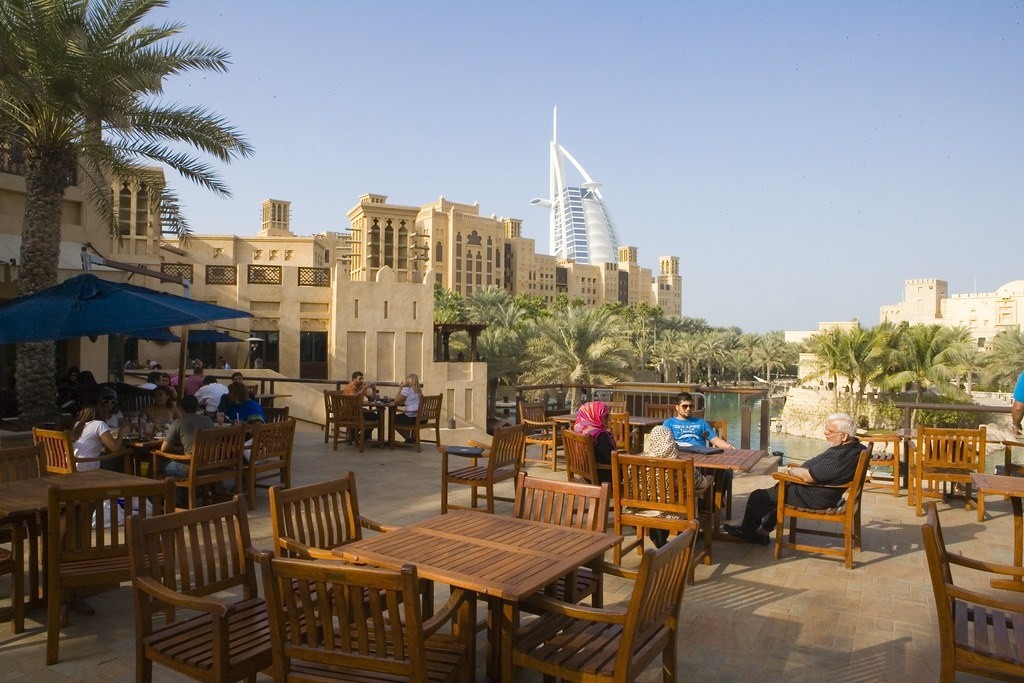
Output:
[120,435,139,439]
[153,434,166,440]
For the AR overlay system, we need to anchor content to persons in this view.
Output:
[146,360,158,369]
[227,382,268,440]
[98,386,125,429]
[162,374,178,400]
[724,413,868,545]
[141,386,182,426]
[192,376,230,415]
[394,374,428,443]
[72,371,100,418]
[69,402,153,528]
[185,367,205,394]
[192,356,231,369]
[250,341,259,369]
[622,424,708,548]
[662,392,735,513]
[68,365,80,385]
[232,371,255,399]
[141,372,161,390]
[147,395,216,478]
[124,358,139,370]
[574,401,625,486]
[342,371,379,441]
[1011,371,1024,436]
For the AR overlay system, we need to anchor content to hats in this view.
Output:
[100,386,117,399]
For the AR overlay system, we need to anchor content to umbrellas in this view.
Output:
[245,338,264,341]
[130,329,247,342]
[1,248,255,407]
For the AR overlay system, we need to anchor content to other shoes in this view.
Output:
[404,438,412,443]
[744,534,770,544]
[724,524,754,541]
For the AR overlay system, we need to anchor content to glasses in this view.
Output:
[679,404,695,410]
[825,426,843,433]
[103,400,117,405]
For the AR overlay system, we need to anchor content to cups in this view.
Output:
[144,423,153,441]
[216,411,225,428]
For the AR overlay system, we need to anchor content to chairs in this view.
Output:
[0,384,1024,683]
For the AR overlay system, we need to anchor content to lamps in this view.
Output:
[245,337,264,352]
